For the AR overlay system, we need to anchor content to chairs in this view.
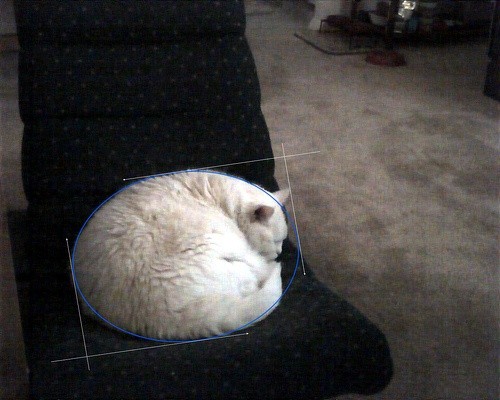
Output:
[12,0,394,400]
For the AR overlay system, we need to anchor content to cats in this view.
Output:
[73,171,290,340]
